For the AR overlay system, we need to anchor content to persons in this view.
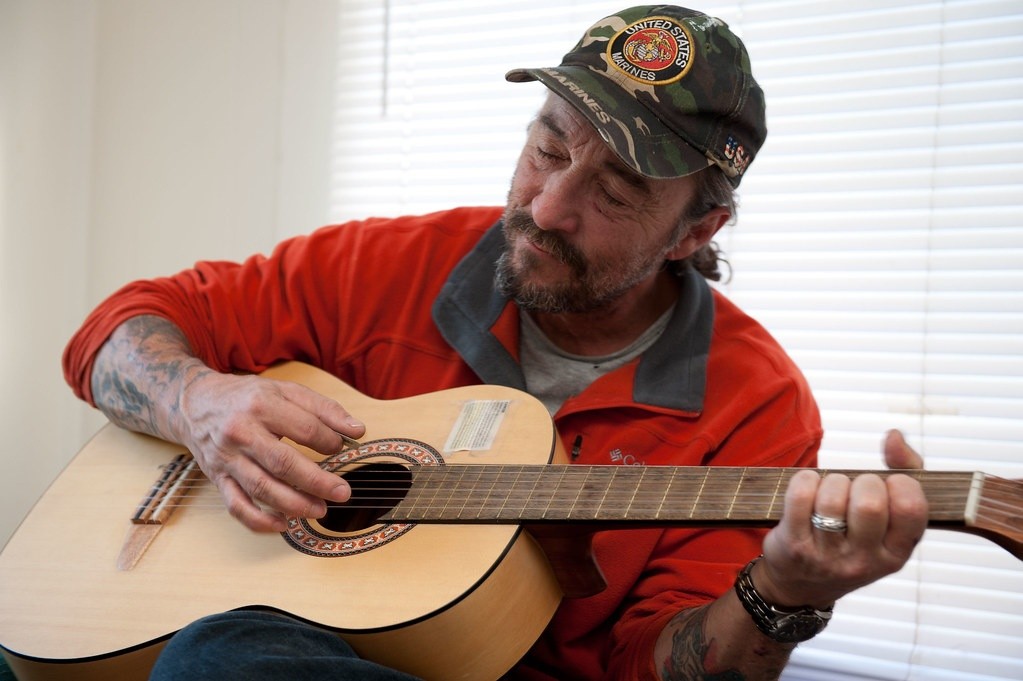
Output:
[61,3,930,681]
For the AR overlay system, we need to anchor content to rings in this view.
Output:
[810,513,847,532]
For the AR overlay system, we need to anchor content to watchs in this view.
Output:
[733,554,833,644]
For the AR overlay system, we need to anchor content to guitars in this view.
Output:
[1,359,1023,681]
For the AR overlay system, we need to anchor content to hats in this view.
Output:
[504,6,768,191]
